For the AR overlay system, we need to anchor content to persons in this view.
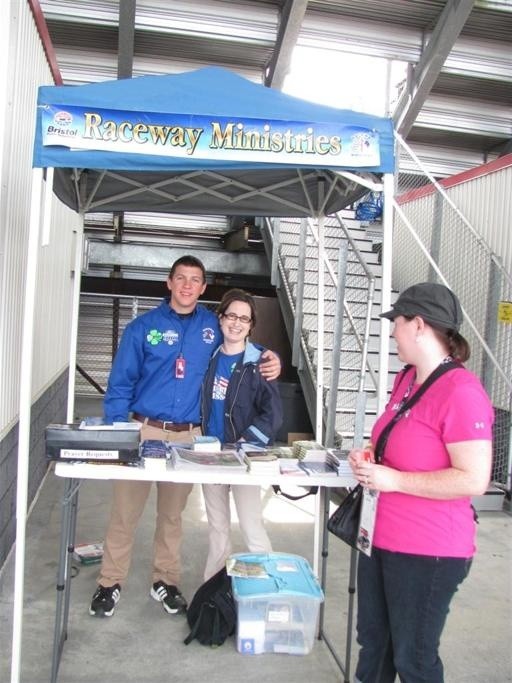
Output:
[196,287,283,584]
[88,253,283,619]
[344,280,495,683]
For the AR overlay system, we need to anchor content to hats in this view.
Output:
[378,282,464,333]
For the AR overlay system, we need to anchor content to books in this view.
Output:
[140,433,358,477]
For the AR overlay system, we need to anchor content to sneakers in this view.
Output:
[150,580,189,616]
[87,581,122,618]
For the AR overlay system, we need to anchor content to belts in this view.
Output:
[130,413,202,433]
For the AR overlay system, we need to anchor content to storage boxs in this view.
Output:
[230,550,327,656]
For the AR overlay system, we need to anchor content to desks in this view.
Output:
[49,446,365,683]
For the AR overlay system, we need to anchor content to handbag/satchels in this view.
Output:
[186,565,236,650]
[326,479,365,552]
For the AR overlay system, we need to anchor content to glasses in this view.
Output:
[224,312,253,325]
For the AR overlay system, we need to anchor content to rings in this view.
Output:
[365,475,370,483]
